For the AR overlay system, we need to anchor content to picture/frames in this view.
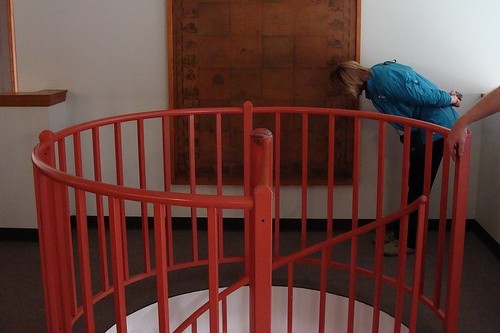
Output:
[167,0,361,184]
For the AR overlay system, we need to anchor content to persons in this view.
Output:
[329,60,462,256]
[446,86,500,161]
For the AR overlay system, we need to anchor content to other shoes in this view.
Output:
[381,240,416,256]
